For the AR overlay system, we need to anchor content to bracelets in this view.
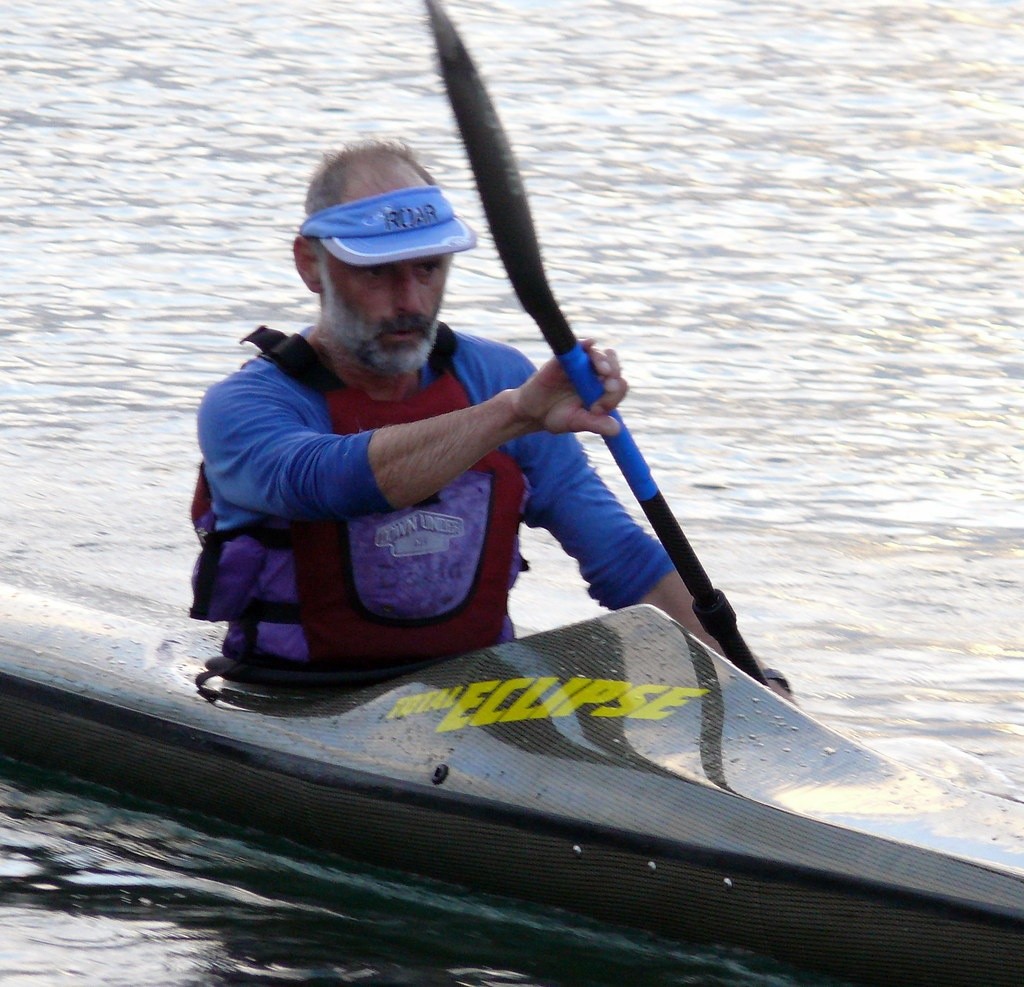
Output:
[761,668,791,693]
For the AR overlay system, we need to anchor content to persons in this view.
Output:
[188,136,795,702]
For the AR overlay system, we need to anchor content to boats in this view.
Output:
[0,559,1024,987]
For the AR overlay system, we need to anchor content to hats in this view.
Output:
[301,186,477,268]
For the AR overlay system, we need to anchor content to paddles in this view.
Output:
[417,0,774,695]
